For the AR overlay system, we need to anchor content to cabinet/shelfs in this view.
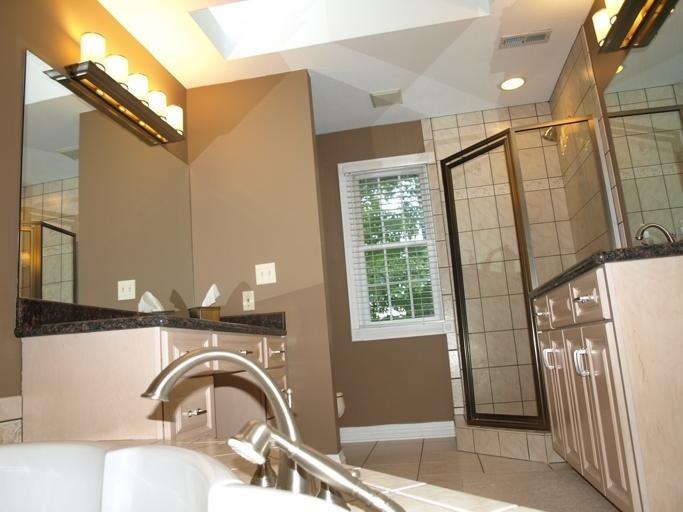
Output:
[531,257,683,512]
[22,327,296,443]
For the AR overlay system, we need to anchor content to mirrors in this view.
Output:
[600,0,683,247]
[18,47,194,317]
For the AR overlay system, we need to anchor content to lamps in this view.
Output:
[65,32,187,142]
[591,1,676,53]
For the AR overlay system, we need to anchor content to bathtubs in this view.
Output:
[0,439,349,512]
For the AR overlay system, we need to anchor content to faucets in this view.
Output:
[138,345,311,495]
[635,224,674,244]
